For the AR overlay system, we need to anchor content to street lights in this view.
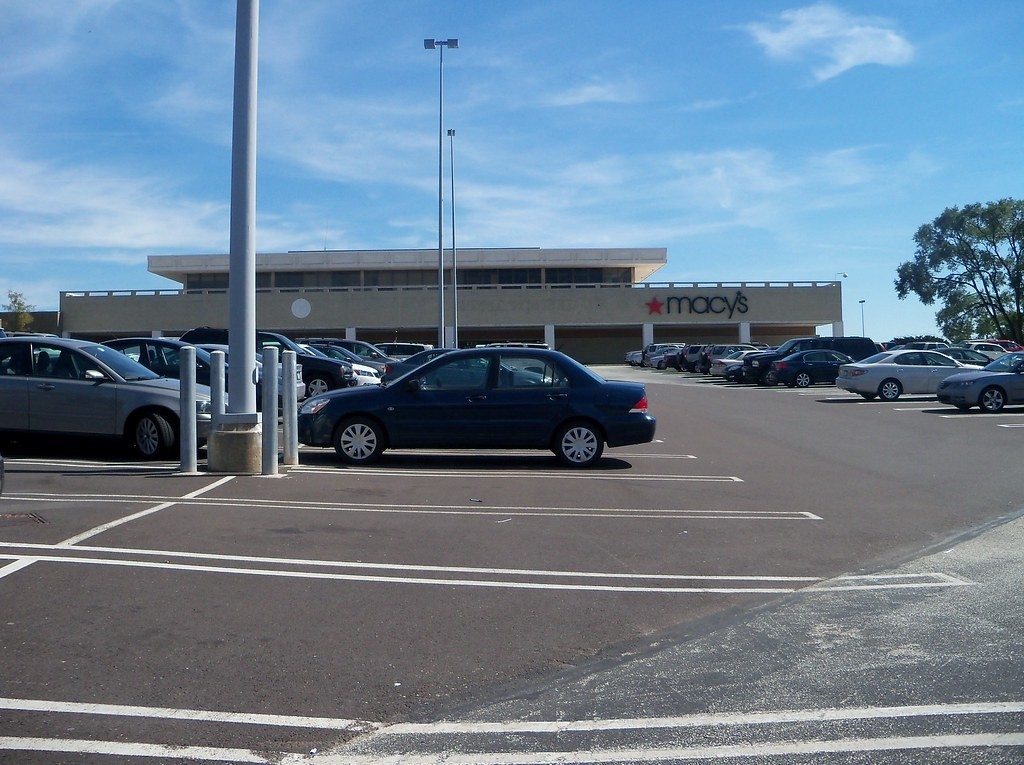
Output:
[423,38,459,349]
[859,299,866,338]
[447,128,458,348]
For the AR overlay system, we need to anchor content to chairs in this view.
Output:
[6,349,32,375]
[56,353,80,380]
[953,353,962,358]
[38,352,50,362]
[149,350,155,360]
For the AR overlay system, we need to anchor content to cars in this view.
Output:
[935,351,1024,414]
[625,336,1024,389]
[0,325,559,413]
[0,336,230,461]
[295,347,657,467]
[836,350,985,402]
